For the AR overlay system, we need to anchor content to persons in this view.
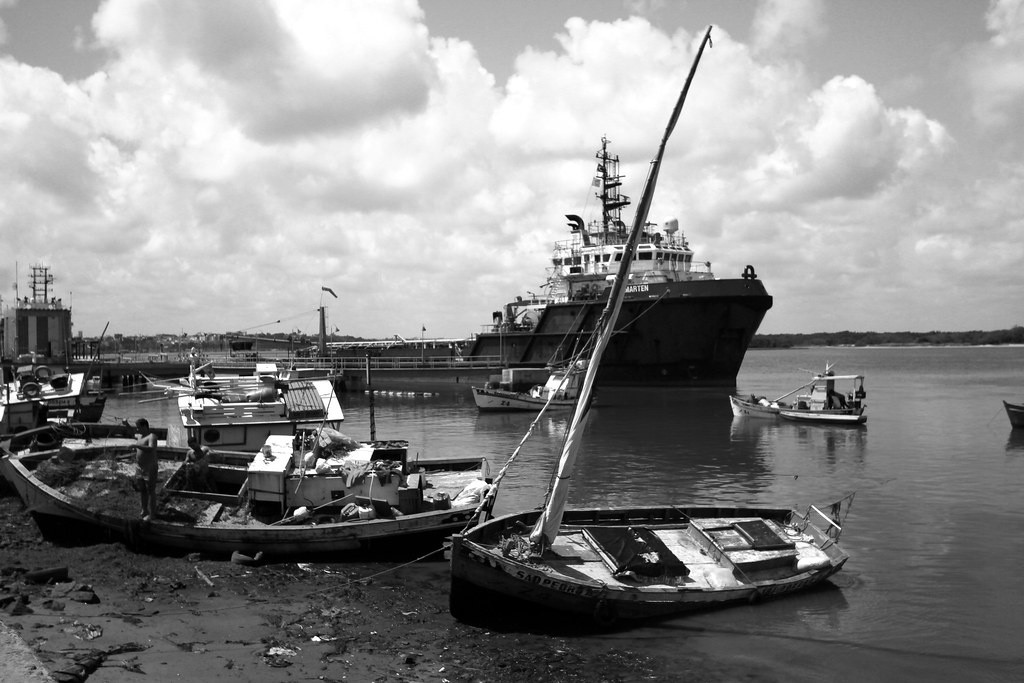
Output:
[749,393,766,404]
[122,417,159,521]
[185,437,210,463]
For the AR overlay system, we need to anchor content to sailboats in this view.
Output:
[446,25,857,636]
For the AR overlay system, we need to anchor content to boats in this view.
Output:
[1003,400,1024,429]
[134,346,344,454]
[779,359,867,425]
[729,361,839,420]
[0,321,112,439]
[485,133,774,387]
[471,296,602,412]
[0,359,493,563]
